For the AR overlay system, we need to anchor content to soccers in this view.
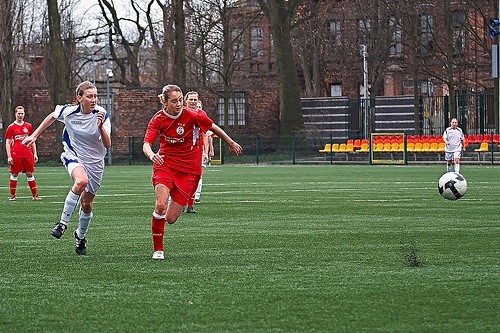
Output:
[437,172,469,201]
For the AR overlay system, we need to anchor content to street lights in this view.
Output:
[93,25,122,165]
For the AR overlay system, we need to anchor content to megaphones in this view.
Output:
[488,17,500,29]
[489,29,500,39]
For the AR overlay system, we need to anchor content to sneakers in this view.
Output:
[73,230,87,254]
[50,223,66,238]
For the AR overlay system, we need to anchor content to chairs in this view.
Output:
[319,134,500,161]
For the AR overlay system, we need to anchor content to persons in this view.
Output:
[196,102,214,156]
[167,91,209,203]
[5,106,42,201]
[143,85,243,260]
[443,118,467,173]
[22,81,111,255]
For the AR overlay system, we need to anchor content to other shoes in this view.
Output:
[187,208,196,212]
[32,196,42,201]
[194,199,201,204]
[9,197,16,200]
[152,250,164,259]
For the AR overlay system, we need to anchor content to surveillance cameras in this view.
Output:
[93,36,101,44]
[114,35,122,44]
[108,72,114,78]
